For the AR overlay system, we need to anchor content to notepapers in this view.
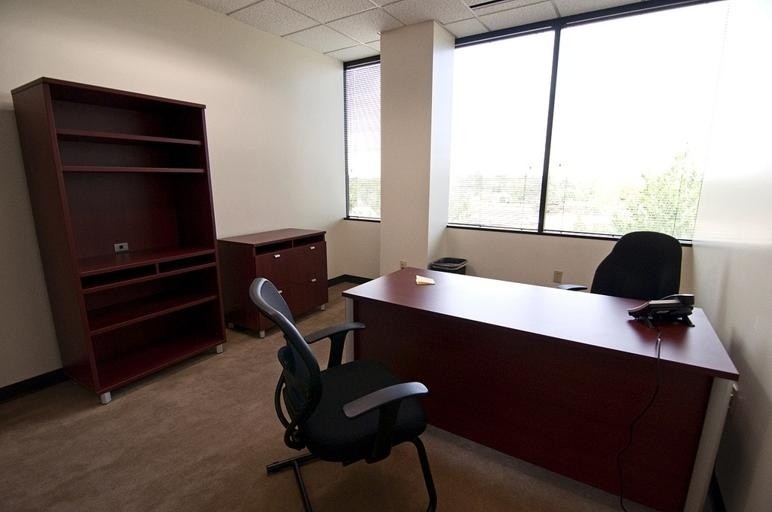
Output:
[415,273,436,286]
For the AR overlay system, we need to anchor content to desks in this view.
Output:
[341,267,740,512]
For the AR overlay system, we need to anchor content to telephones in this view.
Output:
[628,294,696,328]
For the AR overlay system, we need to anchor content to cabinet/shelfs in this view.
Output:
[10,75,229,405]
[218,228,329,339]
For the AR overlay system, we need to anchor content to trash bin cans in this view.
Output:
[431,258,467,275]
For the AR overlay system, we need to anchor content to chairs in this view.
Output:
[249,277,438,511]
[555,231,682,300]
